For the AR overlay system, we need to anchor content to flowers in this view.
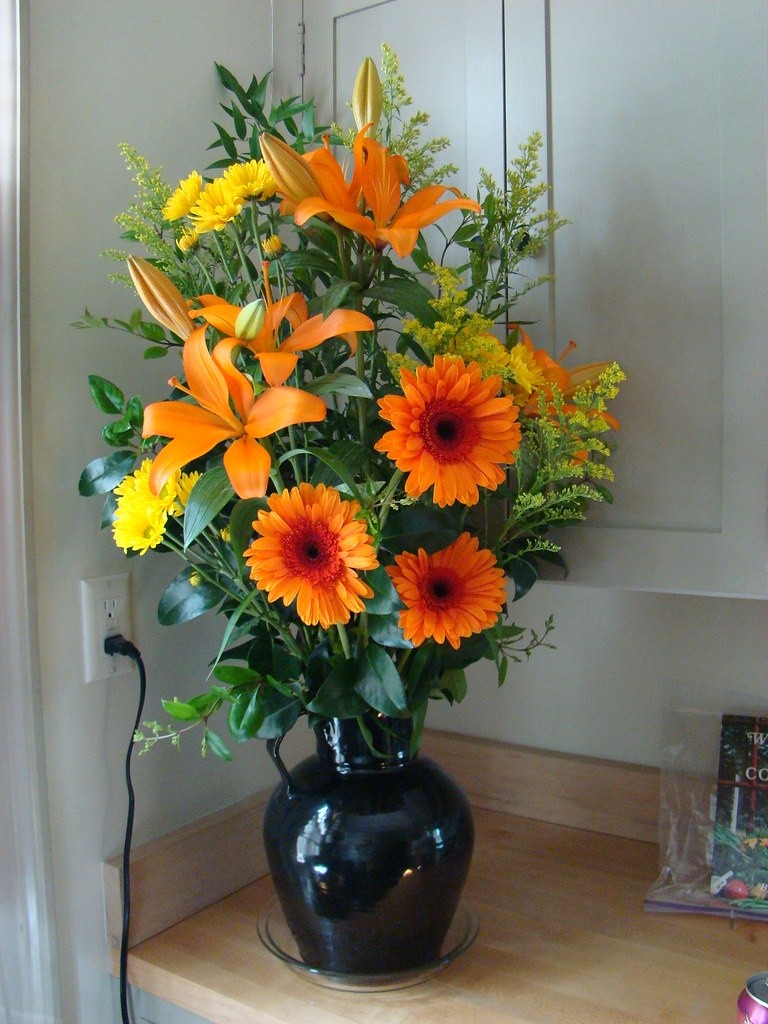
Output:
[68,36,627,763]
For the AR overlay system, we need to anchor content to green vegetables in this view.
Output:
[710,823,768,890]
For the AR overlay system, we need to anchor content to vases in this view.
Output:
[262,711,476,989]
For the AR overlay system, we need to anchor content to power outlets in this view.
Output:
[80,572,135,684]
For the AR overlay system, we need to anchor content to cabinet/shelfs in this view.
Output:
[301,0,768,601]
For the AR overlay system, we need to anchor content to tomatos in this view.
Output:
[724,879,749,899]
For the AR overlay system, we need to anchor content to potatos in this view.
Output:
[748,886,767,899]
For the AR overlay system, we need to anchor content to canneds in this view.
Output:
[736,971,768,1024]
